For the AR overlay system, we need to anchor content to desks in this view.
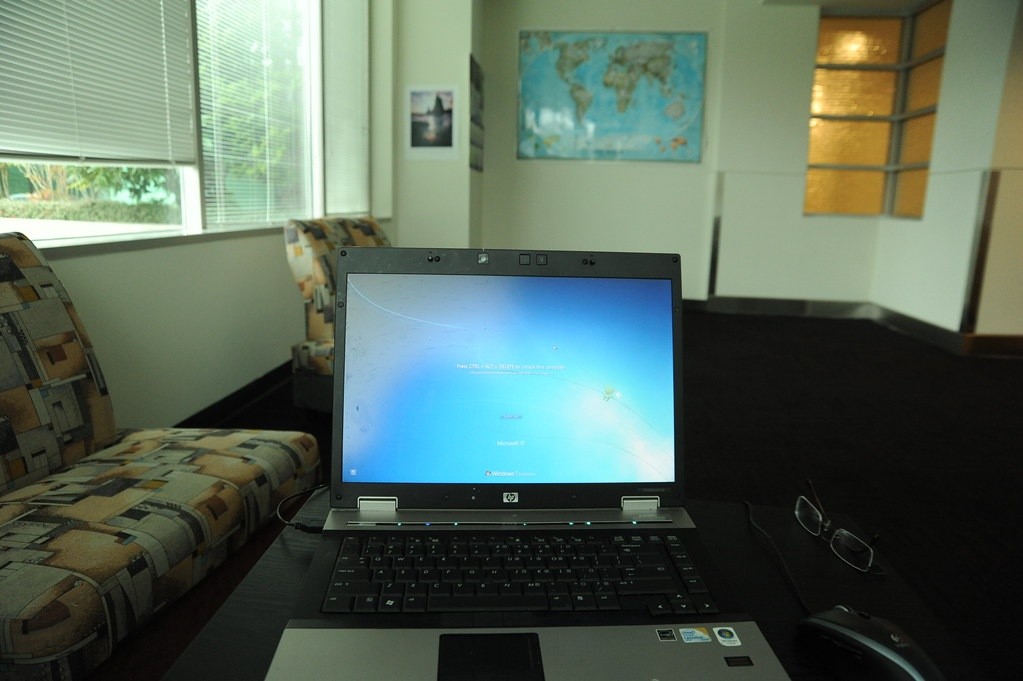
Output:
[166,485,979,680]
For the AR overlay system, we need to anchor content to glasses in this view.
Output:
[786,477,889,576]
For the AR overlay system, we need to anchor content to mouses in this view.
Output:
[807,604,944,681]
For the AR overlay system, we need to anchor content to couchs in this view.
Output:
[286,219,389,415]
[0,233,323,680]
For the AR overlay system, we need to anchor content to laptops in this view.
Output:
[263,247,803,681]
